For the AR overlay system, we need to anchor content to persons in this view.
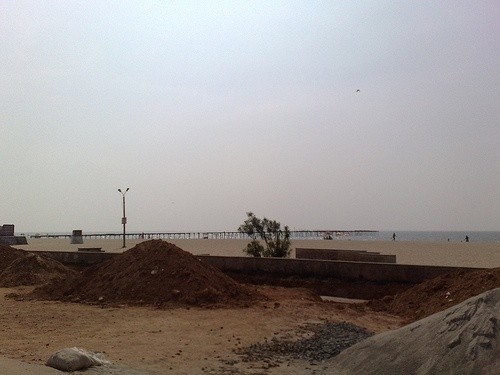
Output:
[323,231,469,243]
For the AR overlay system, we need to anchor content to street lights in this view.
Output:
[117,188,129,248]
